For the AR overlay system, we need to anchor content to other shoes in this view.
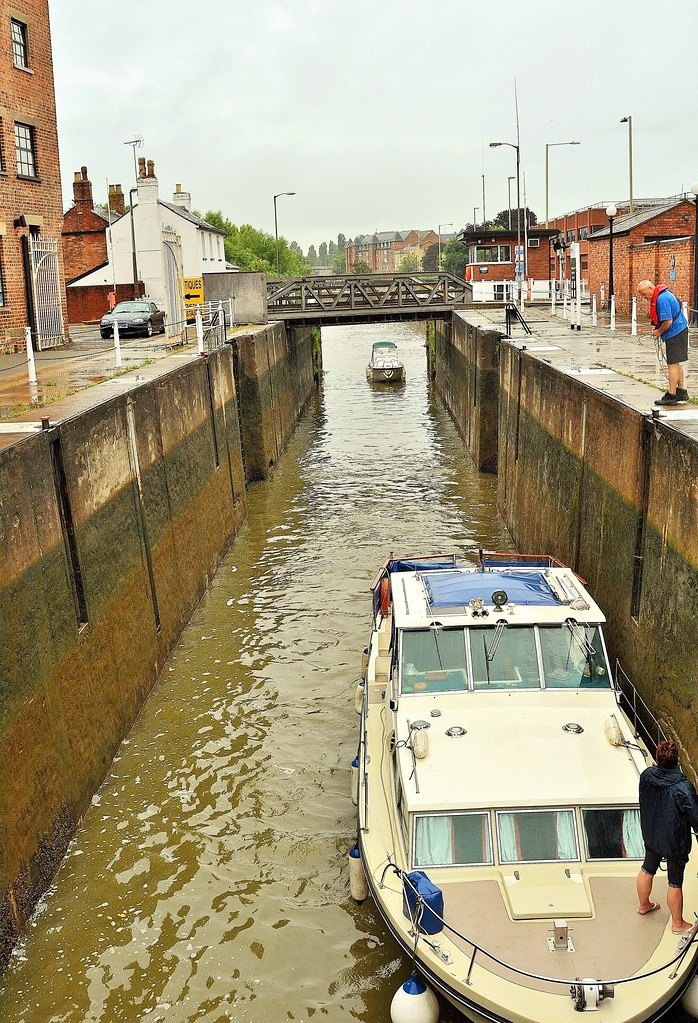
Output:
[654,387,689,406]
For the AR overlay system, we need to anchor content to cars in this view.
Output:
[97,300,165,338]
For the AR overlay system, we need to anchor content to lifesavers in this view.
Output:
[379,577,389,620]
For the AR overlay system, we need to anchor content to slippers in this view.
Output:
[672,922,697,935]
[637,902,660,914]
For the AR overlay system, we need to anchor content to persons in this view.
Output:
[637,279,690,406]
[636,740,698,934]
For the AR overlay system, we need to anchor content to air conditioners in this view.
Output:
[528,239,539,248]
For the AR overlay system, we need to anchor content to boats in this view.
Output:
[349,547,697,1022]
[368,341,405,383]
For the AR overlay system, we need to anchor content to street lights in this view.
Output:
[507,176,516,229]
[438,223,453,272]
[691,180,698,328]
[489,143,521,306]
[129,188,139,298]
[545,141,581,229]
[482,175,486,231]
[605,205,618,313]
[274,192,295,278]
[620,115,634,216]
[474,207,480,231]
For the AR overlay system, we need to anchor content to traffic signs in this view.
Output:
[178,275,205,320]
